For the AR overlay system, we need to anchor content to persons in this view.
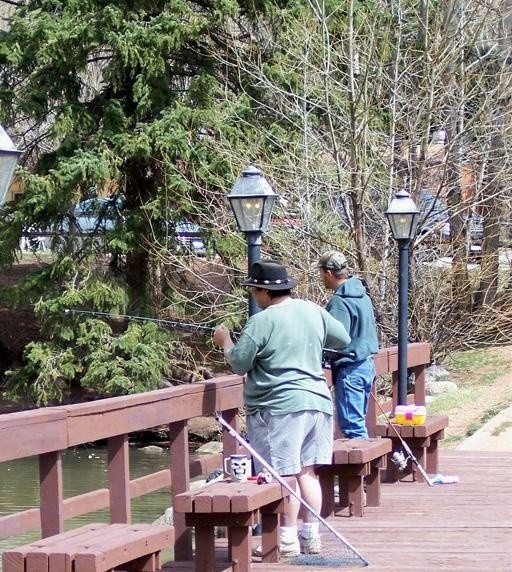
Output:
[212,257,353,556]
[318,251,379,437]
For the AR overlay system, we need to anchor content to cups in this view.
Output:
[224,455,249,484]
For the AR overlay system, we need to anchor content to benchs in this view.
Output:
[374,413,449,484]
[0,519,178,572]
[313,435,395,520]
[172,476,298,572]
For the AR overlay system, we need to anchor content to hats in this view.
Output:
[238,257,298,291]
[311,250,348,271]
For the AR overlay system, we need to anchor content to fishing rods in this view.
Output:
[369,390,433,487]
[63,309,355,368]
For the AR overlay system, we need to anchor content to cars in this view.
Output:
[330,190,483,263]
[17,198,209,257]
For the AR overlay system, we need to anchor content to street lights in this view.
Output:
[385,187,421,406]
[227,164,280,317]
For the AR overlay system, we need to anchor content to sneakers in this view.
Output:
[297,534,322,554]
[249,539,301,563]
